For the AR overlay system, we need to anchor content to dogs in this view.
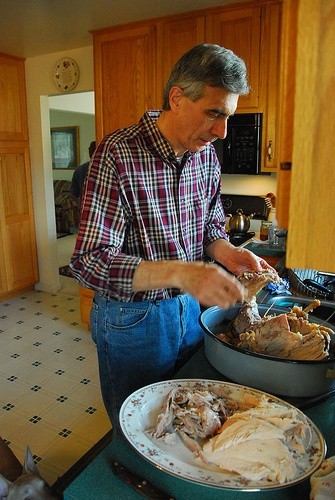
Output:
[0,446,57,500]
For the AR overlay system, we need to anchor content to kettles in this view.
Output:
[225,209,256,235]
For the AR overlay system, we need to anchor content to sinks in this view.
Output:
[268,295,335,330]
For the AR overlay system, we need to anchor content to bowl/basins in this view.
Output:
[197,302,335,398]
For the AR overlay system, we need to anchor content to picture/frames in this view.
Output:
[50,126,80,170]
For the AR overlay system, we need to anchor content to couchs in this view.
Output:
[55,180,80,233]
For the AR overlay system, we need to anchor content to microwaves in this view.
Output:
[212,114,271,176]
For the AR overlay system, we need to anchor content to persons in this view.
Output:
[70,141,96,222]
[69,43,277,427]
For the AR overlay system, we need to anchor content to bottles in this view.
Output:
[260,221,272,242]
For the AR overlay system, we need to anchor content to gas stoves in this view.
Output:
[220,194,270,248]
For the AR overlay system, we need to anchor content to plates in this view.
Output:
[118,377,327,493]
[52,56,79,92]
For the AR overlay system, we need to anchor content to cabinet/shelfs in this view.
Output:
[83,0,335,333]
[0,51,42,302]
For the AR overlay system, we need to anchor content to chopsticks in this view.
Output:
[110,459,179,500]
